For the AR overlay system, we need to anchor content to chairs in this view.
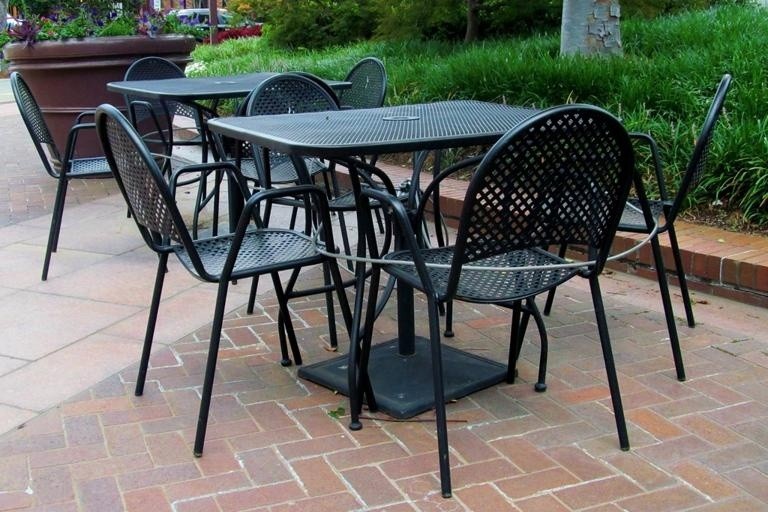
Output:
[10,55,388,282]
[355,104,635,499]
[247,73,398,367]
[517,73,733,383]
[95,104,379,459]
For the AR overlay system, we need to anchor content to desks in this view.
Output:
[207,99,541,419]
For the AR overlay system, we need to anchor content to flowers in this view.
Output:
[1,1,216,52]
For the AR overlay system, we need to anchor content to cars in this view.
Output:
[176,8,265,44]
